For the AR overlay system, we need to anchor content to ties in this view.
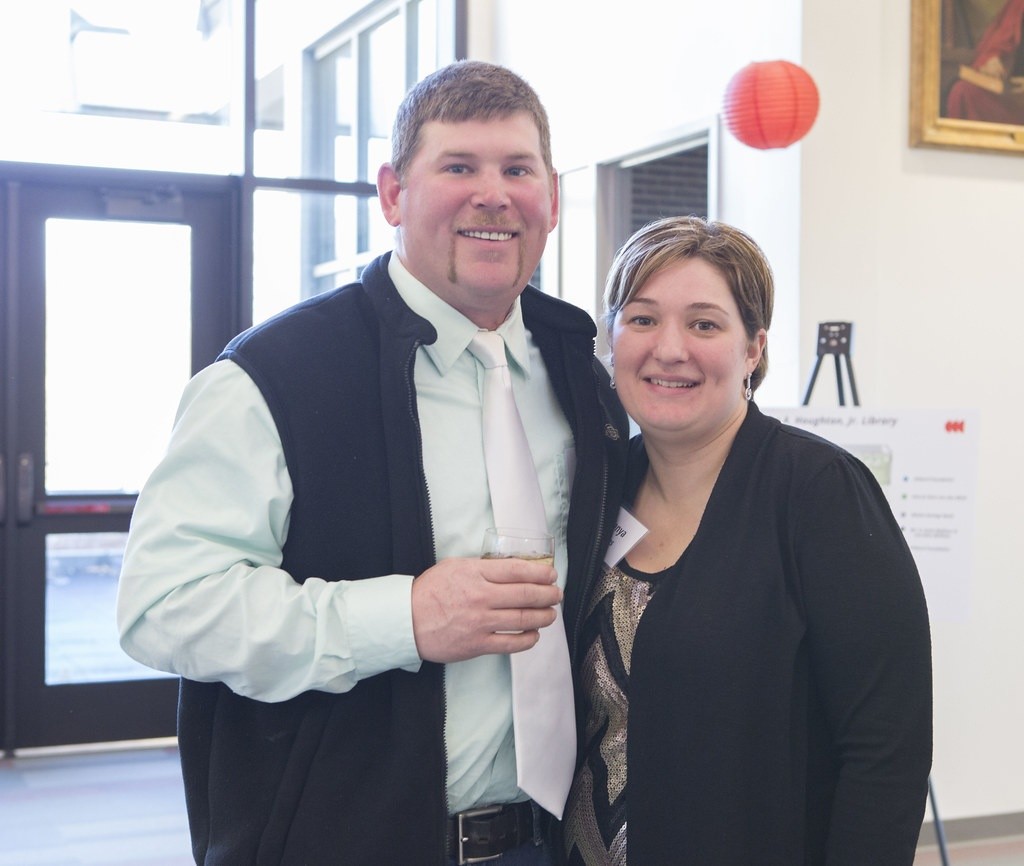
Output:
[465,328,578,820]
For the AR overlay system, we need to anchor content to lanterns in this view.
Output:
[723,60,820,149]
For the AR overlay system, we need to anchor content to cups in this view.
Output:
[481,526,555,568]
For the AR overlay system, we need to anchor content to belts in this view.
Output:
[446,800,551,866]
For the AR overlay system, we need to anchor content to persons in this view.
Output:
[561,217,933,866]
[117,59,630,866]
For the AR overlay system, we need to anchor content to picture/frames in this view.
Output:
[909,0,1024,155]
